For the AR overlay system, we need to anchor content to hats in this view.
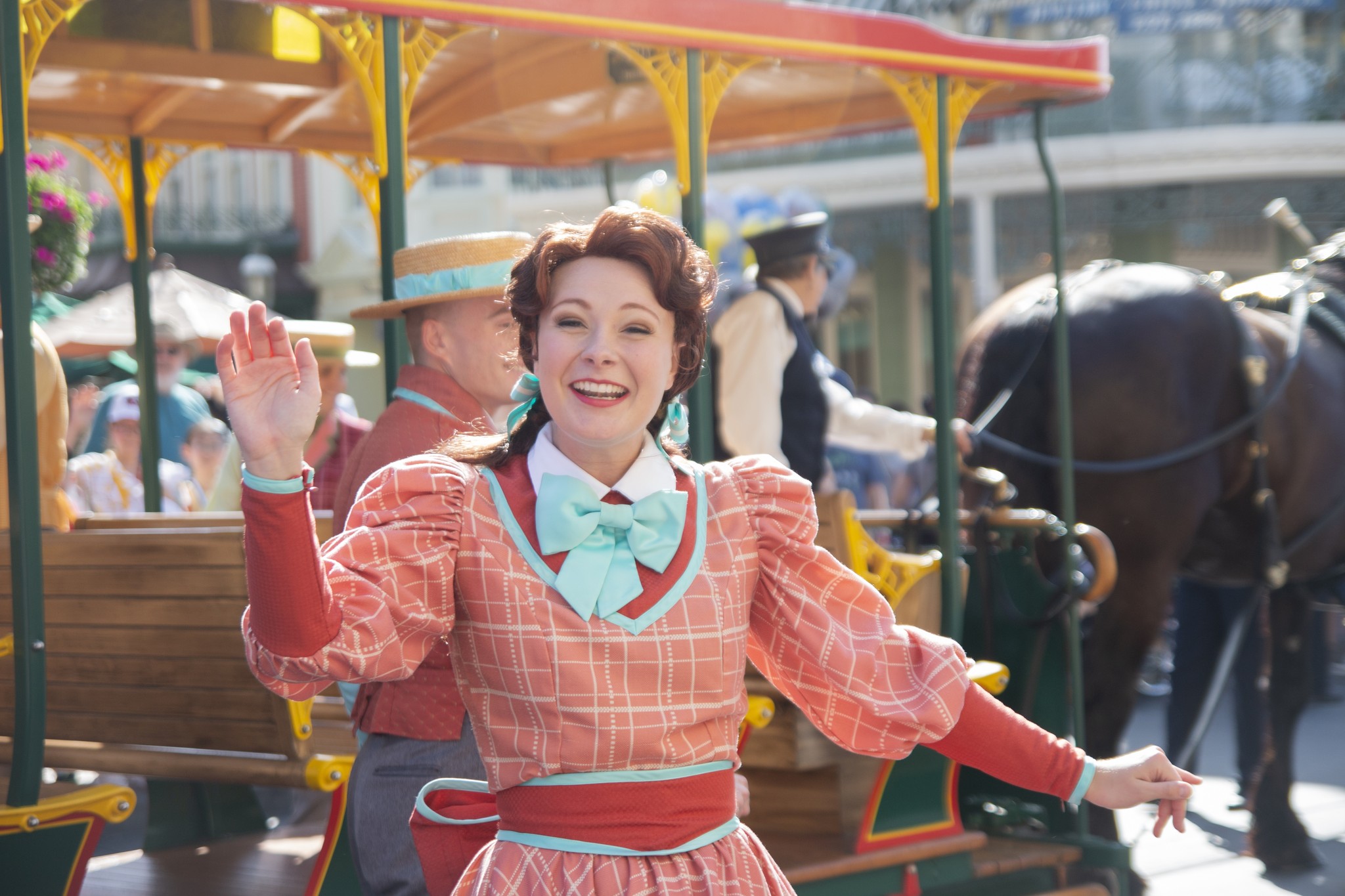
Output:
[349,230,536,316]
[108,387,140,422]
[281,320,381,368]
[745,213,834,265]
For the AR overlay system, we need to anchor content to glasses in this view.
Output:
[814,252,834,280]
[154,347,182,356]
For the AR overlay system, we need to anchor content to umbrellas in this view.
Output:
[39,258,289,364]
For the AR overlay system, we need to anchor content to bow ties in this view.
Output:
[530,472,690,624]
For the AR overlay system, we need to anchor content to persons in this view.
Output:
[0,310,374,856]
[704,211,975,544]
[215,204,1202,896]
[332,230,533,896]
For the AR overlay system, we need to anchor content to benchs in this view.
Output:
[1,512,1011,854]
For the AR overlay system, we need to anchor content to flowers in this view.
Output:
[26,148,107,302]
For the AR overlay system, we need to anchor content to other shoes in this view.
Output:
[1137,659,1172,696]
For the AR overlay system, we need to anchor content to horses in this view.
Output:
[955,229,1345,873]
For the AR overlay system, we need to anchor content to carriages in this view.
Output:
[0,0,1345,896]
